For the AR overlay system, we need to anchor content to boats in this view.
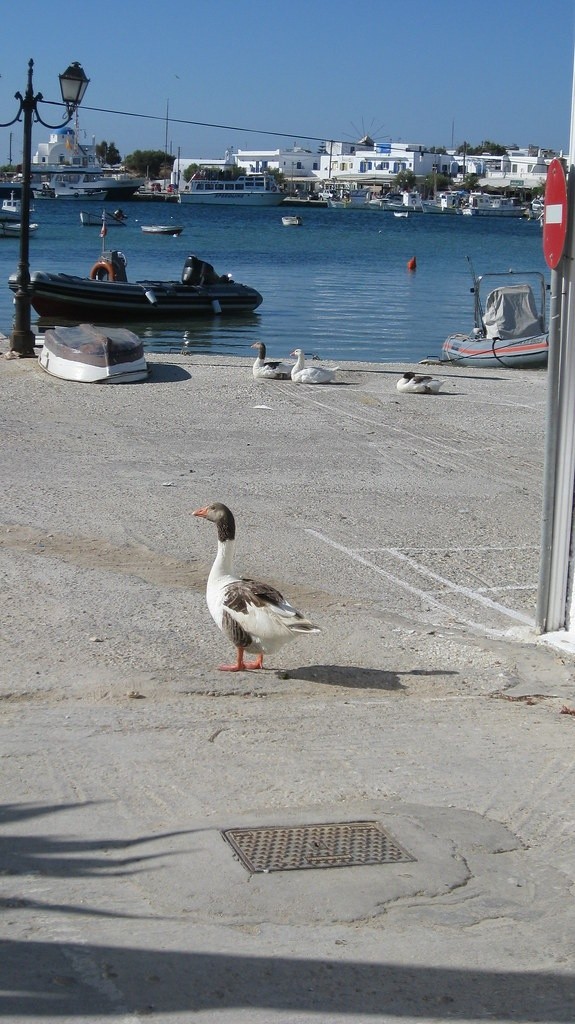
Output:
[421,190,527,216]
[394,211,409,218]
[441,254,549,368]
[37,322,149,384]
[0,191,35,216]
[141,224,184,236]
[80,208,127,226]
[178,170,291,206]
[0,153,148,202]
[364,190,422,211]
[326,188,352,209]
[7,209,263,320]
[282,216,303,226]
[33,182,109,201]
[0,220,38,239]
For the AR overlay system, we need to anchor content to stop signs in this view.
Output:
[543,157,568,271]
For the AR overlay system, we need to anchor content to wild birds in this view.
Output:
[396,372,447,394]
[250,340,295,380]
[289,348,341,384]
[191,500,324,672]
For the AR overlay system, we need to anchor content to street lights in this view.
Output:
[0,57,91,358]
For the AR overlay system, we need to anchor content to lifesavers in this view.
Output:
[89,262,114,281]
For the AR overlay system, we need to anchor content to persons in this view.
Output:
[154,184,159,194]
[43,184,49,190]
[461,199,464,207]
[167,185,172,192]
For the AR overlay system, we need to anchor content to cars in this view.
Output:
[297,191,319,200]
[531,197,544,209]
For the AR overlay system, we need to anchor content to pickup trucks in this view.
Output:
[318,189,340,201]
